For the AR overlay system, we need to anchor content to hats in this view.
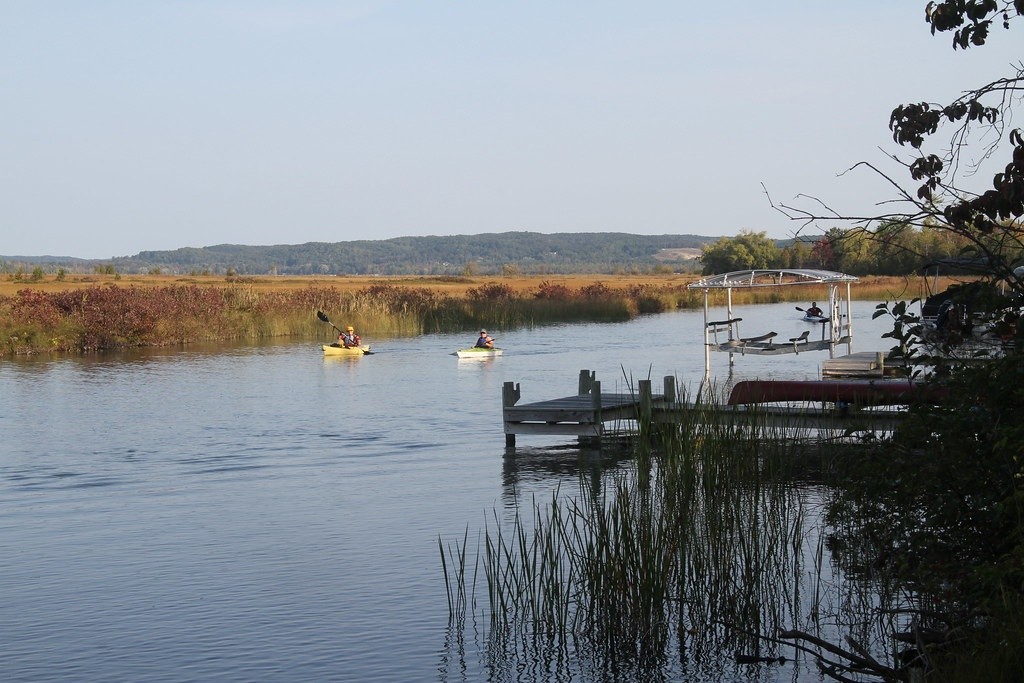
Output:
[480,329,486,333]
[346,327,354,331]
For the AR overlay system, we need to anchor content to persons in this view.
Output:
[337,327,361,349]
[808,302,823,317]
[475,329,494,349]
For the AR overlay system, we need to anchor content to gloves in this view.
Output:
[340,331,344,335]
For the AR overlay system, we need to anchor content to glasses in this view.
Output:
[481,332,486,334]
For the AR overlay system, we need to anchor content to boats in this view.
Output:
[457,348,503,358]
[322,344,370,356]
[801,314,829,323]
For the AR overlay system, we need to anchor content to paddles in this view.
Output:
[474,332,506,347]
[316,310,374,355]
[795,306,821,317]
